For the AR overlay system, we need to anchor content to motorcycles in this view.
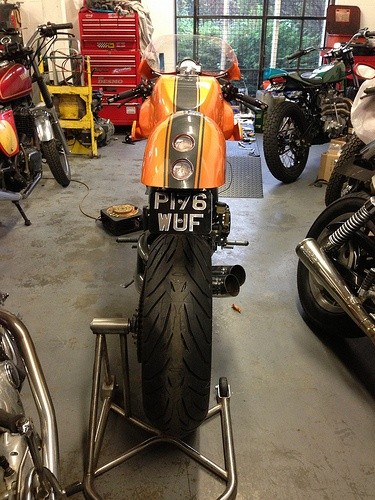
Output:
[107,34,270,435]
[296,160,375,362]
[1,22,74,220]
[325,62,375,203]
[263,27,375,183]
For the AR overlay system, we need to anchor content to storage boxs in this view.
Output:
[318,154,340,184]
